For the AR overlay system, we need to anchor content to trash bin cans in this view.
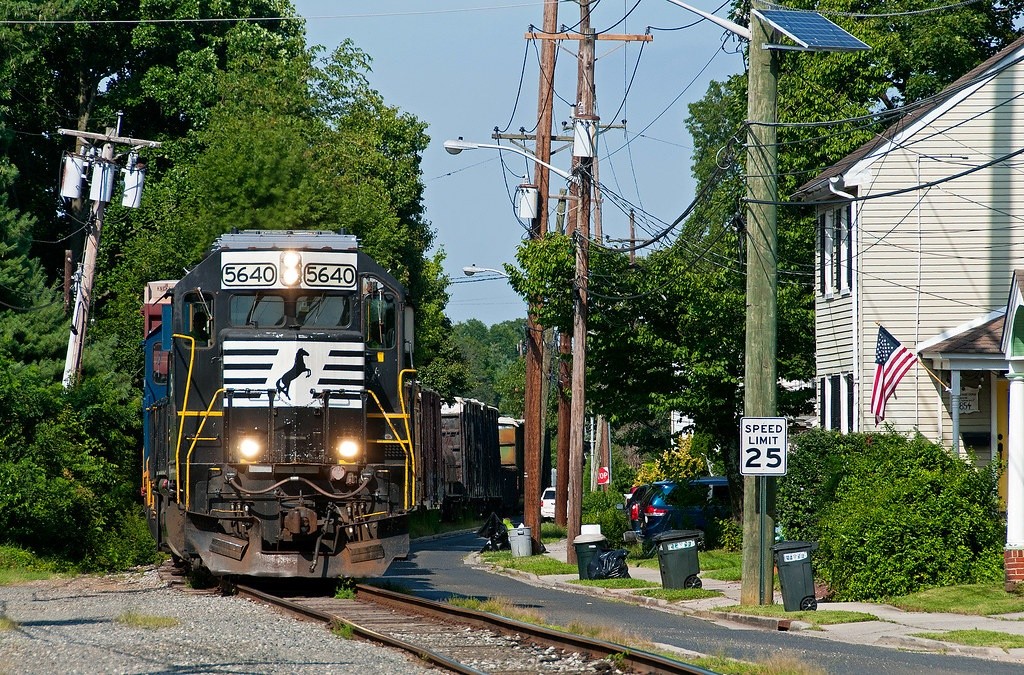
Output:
[508,527,532,557]
[572,533,609,579]
[773,541,821,613]
[653,529,702,589]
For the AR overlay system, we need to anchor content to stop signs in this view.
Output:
[597,467,609,484]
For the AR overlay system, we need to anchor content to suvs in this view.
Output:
[624,477,741,551]
[542,486,572,523]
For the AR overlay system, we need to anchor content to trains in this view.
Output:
[140,230,555,595]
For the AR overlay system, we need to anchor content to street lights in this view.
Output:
[462,262,511,280]
[443,135,587,567]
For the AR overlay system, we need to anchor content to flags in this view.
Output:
[871,325,918,427]
